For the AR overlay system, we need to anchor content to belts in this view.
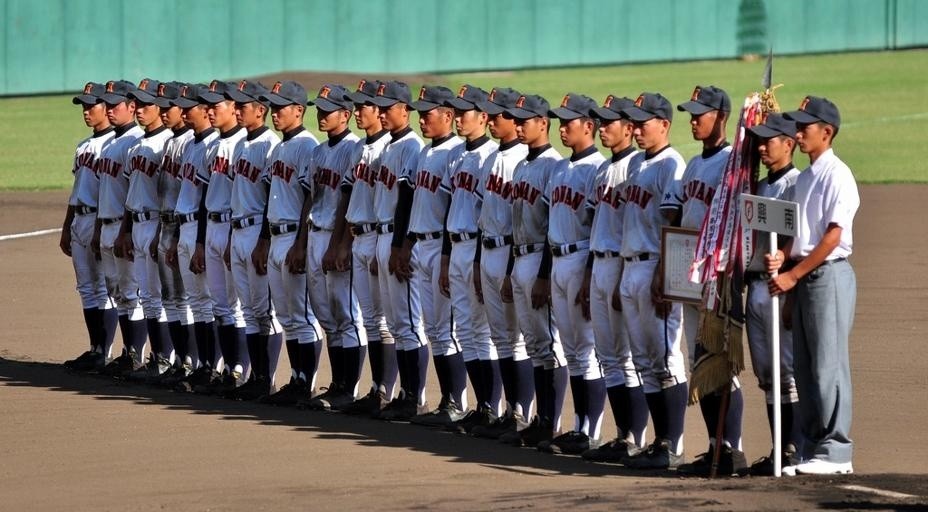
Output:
[790,258,844,266]
[748,271,772,281]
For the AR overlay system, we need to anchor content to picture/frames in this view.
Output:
[658,226,704,306]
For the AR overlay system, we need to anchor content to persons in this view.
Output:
[766,95,861,476]
[60,79,804,479]
